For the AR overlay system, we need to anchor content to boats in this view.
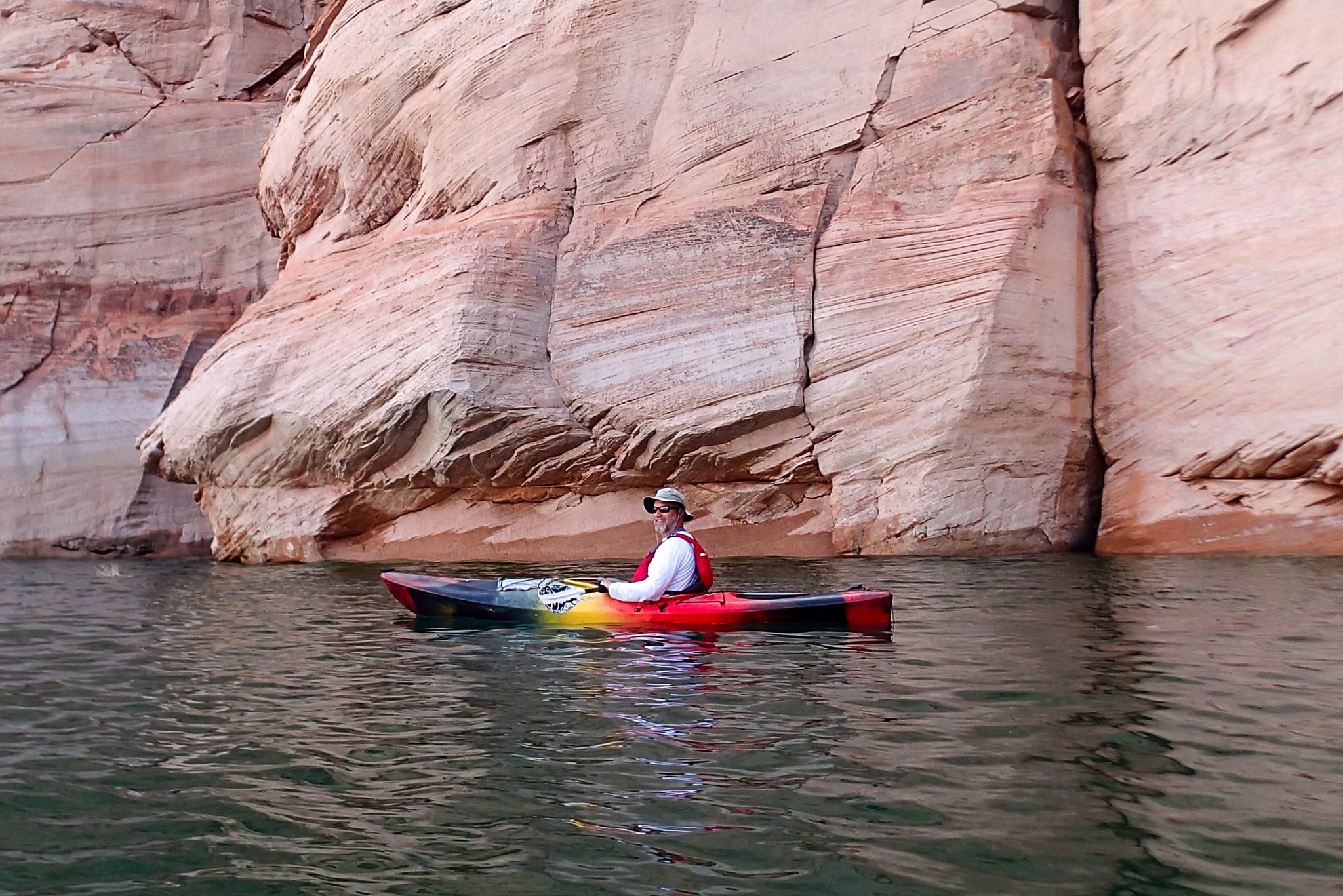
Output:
[380,570,896,632]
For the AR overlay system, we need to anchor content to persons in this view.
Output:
[600,487,704,602]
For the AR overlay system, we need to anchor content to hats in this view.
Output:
[643,487,694,522]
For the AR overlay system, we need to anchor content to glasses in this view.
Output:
[651,505,680,513]
